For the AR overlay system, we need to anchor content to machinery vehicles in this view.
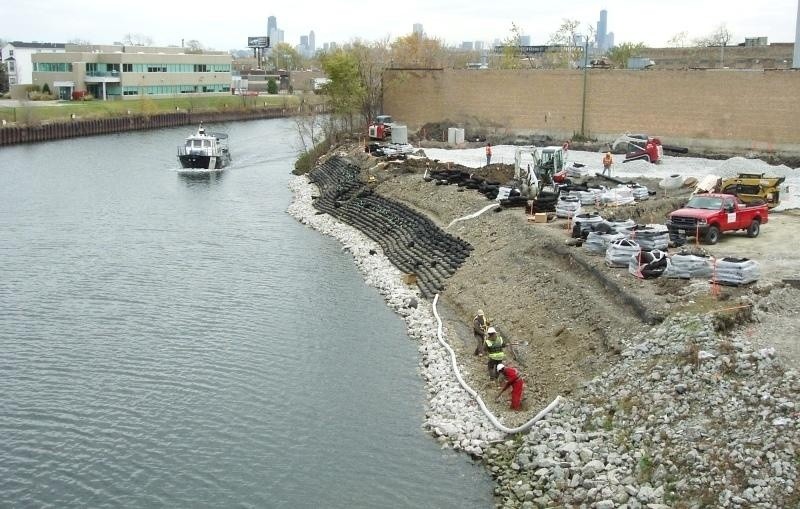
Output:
[367,115,397,141]
[713,172,789,212]
[509,143,572,195]
[611,133,665,164]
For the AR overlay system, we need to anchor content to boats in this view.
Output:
[175,120,231,173]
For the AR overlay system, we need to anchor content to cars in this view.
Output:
[240,90,259,96]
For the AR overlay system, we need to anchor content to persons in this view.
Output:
[563,141,569,164]
[496,363,523,411]
[473,309,491,356]
[484,326,509,380]
[486,143,492,165]
[602,152,612,178]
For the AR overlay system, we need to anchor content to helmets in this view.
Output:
[487,327,496,334]
[477,309,485,316]
[497,364,504,374]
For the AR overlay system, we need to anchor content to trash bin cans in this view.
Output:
[232,88,236,95]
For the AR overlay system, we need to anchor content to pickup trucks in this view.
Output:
[586,58,615,69]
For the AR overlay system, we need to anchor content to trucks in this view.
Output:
[625,56,656,69]
[663,192,772,246]
[308,77,333,95]
